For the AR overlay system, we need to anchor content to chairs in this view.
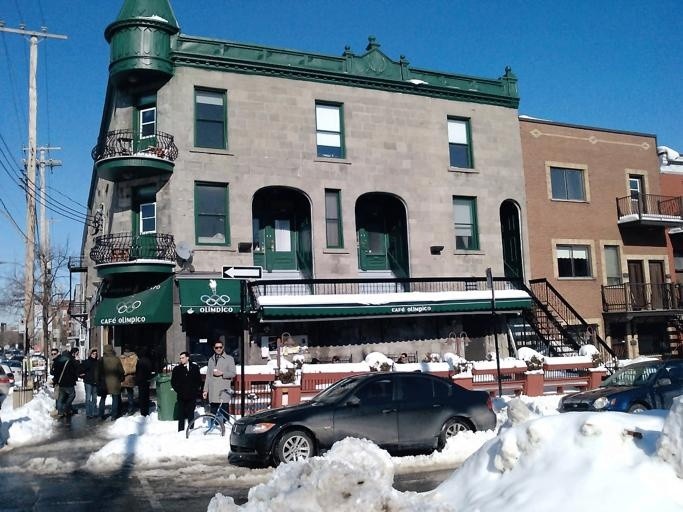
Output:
[649,367,679,383]
[368,382,393,402]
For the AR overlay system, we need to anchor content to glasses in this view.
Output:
[215,346,222,349]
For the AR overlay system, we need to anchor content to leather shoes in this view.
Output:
[128,407,138,416]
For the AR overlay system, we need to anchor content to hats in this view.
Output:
[89,348,98,357]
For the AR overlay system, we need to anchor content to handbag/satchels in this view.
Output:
[51,384,60,400]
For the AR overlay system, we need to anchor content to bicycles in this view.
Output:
[186,389,259,438]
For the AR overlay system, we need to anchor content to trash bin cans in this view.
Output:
[156,373,186,421]
[13,387,34,409]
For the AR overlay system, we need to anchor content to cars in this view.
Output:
[556,360,683,413]
[228,370,497,468]
[0,351,24,396]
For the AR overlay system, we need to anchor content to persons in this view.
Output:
[51,347,150,425]
[203,340,236,425]
[170,351,201,431]
[332,356,340,363]
[395,353,407,364]
